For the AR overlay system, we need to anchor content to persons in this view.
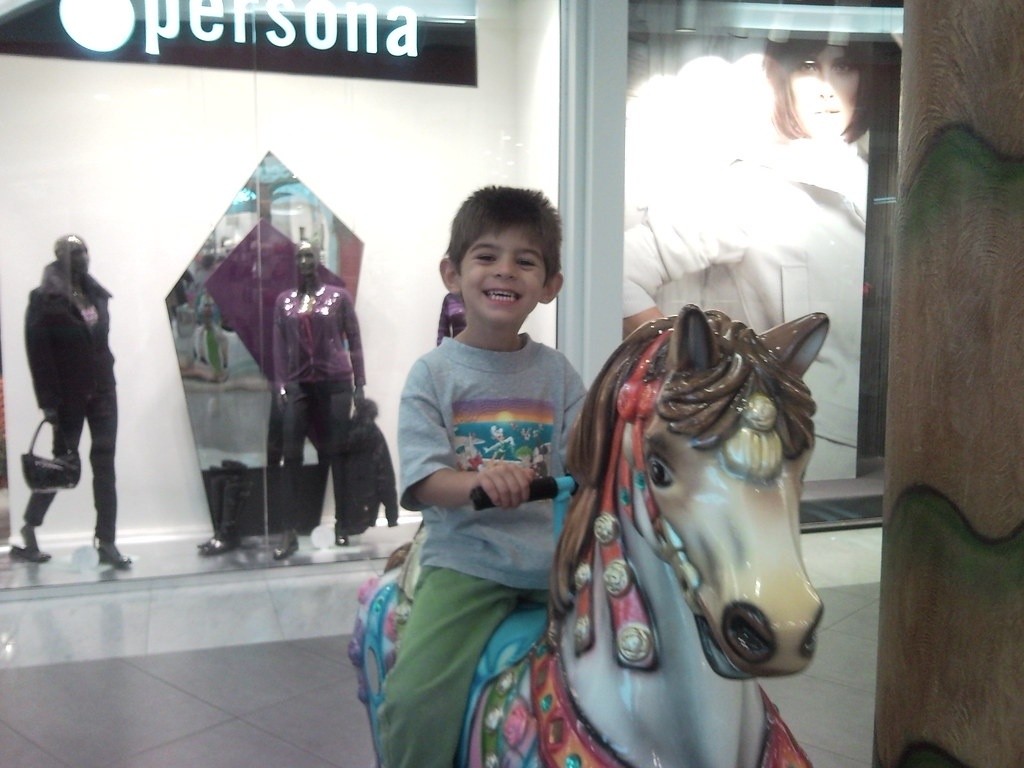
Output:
[436,290,467,345]
[10,233,133,565]
[273,237,367,560]
[376,187,587,768]
[620,26,903,482]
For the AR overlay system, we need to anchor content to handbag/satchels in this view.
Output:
[22,419,81,489]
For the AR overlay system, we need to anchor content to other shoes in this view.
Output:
[274,541,298,559]
[336,535,347,545]
[98,548,130,568]
[9,546,51,562]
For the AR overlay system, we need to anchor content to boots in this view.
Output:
[197,471,224,549]
[203,473,240,554]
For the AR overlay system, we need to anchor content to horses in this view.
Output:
[345,304,833,768]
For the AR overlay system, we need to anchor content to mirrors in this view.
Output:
[164,150,364,549]
